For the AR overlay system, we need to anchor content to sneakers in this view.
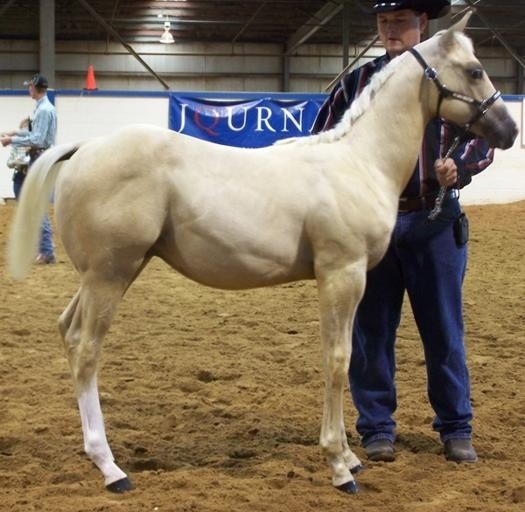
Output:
[364,439,394,462]
[443,437,477,463]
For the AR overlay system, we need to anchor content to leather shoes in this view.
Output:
[32,253,55,264]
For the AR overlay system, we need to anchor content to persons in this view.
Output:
[307,1,496,460]
[6,119,31,201]
[0,76,58,265]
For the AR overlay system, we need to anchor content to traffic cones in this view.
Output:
[83,64,97,91]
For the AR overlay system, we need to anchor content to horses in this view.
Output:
[6,7,521,496]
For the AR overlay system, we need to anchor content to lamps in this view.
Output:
[160,21,175,43]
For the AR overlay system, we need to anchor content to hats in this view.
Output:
[368,1,450,20]
[23,74,46,88]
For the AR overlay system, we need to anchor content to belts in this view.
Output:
[398,190,460,211]
